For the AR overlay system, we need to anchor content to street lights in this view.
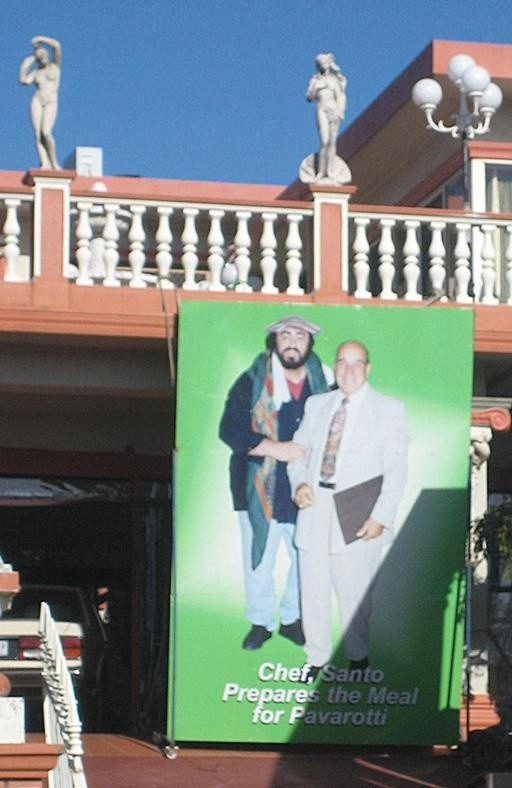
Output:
[465,438,491,701]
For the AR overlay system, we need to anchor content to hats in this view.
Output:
[269,316,319,338]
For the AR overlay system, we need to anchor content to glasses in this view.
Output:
[335,359,364,373]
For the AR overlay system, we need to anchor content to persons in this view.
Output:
[219,314,339,651]
[287,339,410,684]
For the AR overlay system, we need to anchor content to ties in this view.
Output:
[321,400,347,483]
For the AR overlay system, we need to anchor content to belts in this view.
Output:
[319,480,335,491]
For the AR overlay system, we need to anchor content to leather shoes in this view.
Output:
[241,625,273,650]
[280,618,305,647]
[348,660,373,684]
[297,663,320,684]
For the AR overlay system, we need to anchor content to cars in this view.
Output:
[0,578,125,703]
